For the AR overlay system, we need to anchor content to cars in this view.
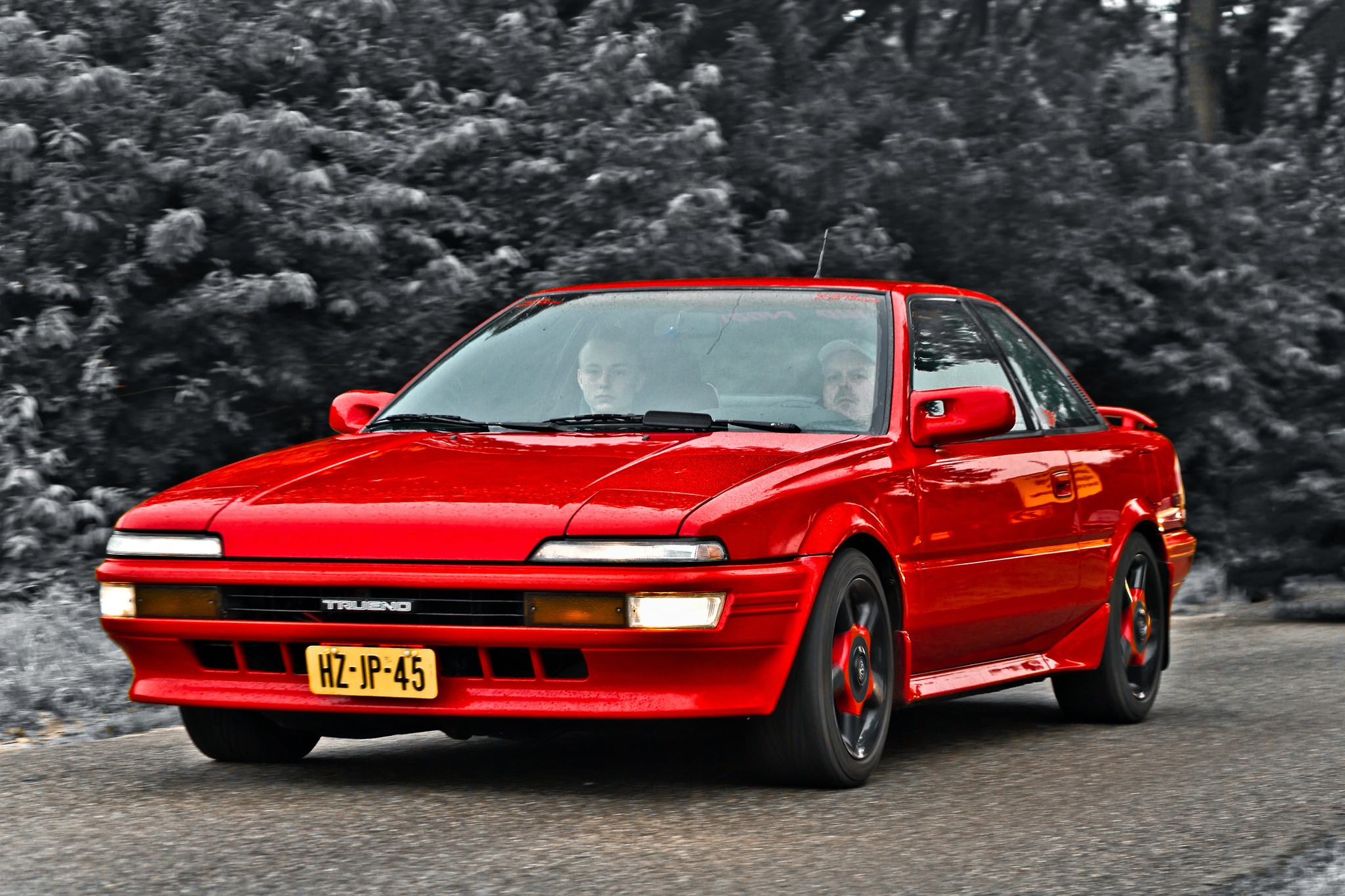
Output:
[97,276,1200,787]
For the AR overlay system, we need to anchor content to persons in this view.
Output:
[577,336,645,413]
[821,336,877,425]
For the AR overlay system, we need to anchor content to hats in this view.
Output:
[817,338,878,373]
